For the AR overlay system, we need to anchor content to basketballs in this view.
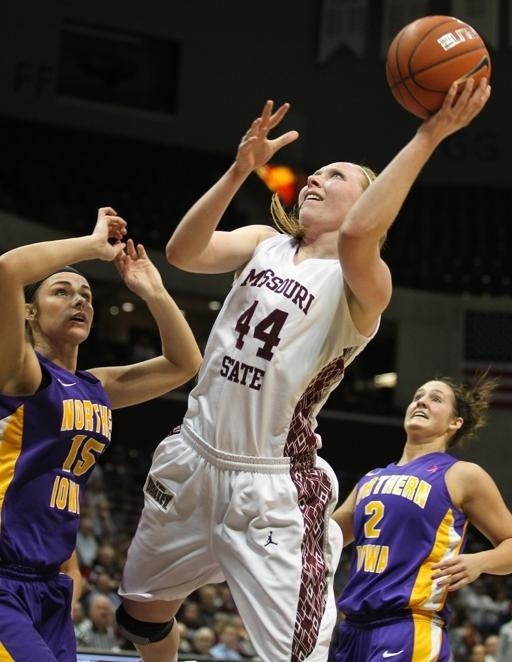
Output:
[386,16,490,120]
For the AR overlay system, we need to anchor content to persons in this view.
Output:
[331,358,511,662]
[61,435,512,662]
[0,203,206,662]
[114,74,495,662]
